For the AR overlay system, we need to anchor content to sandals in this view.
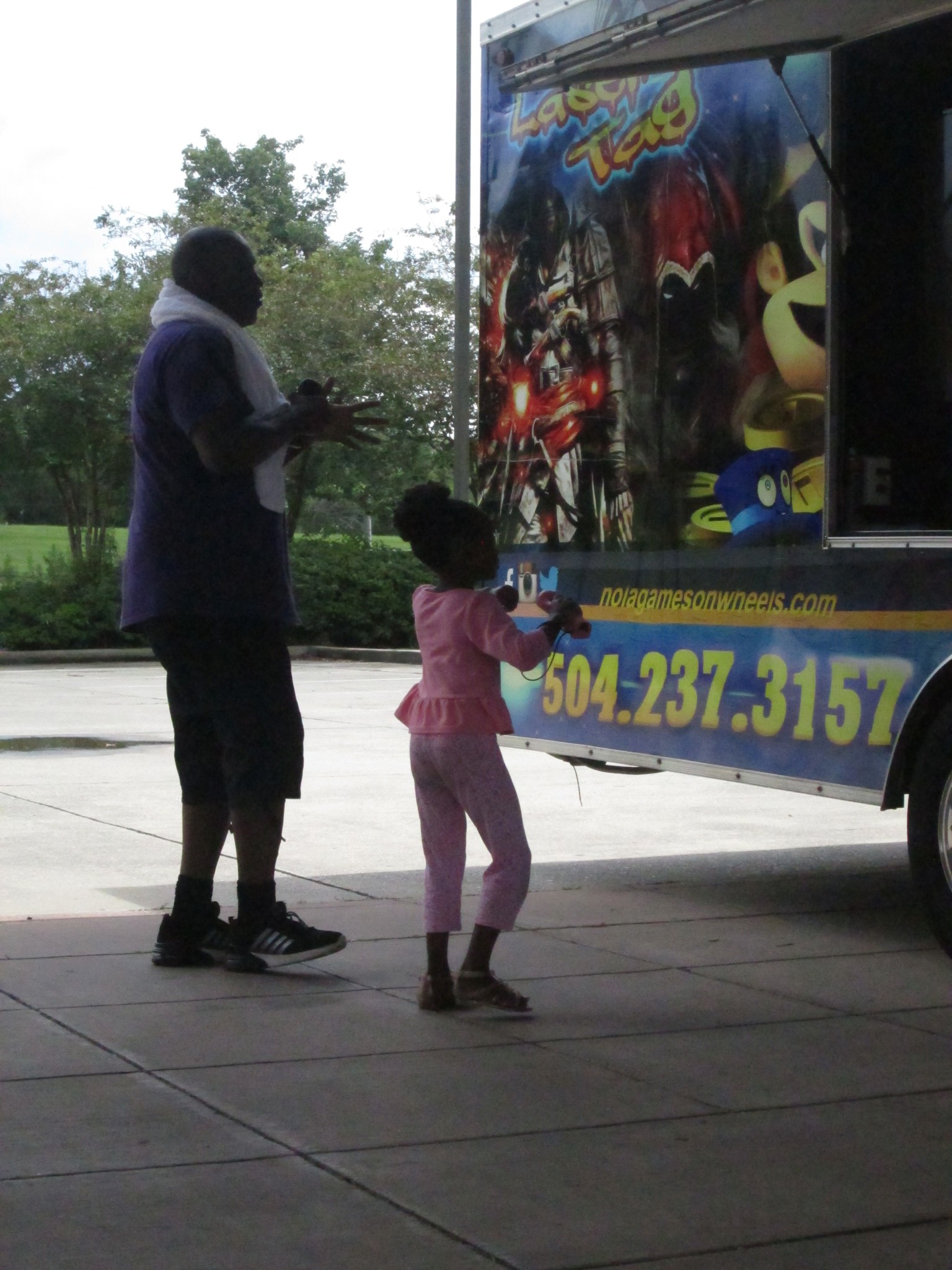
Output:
[416,970,454,1010]
[453,969,533,1013]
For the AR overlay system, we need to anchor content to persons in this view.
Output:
[393,473,586,1015]
[120,227,391,976]
[473,180,637,551]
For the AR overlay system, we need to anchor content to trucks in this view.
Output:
[454,0,951,953]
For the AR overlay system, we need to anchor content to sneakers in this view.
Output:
[228,900,347,968]
[151,901,269,971]
